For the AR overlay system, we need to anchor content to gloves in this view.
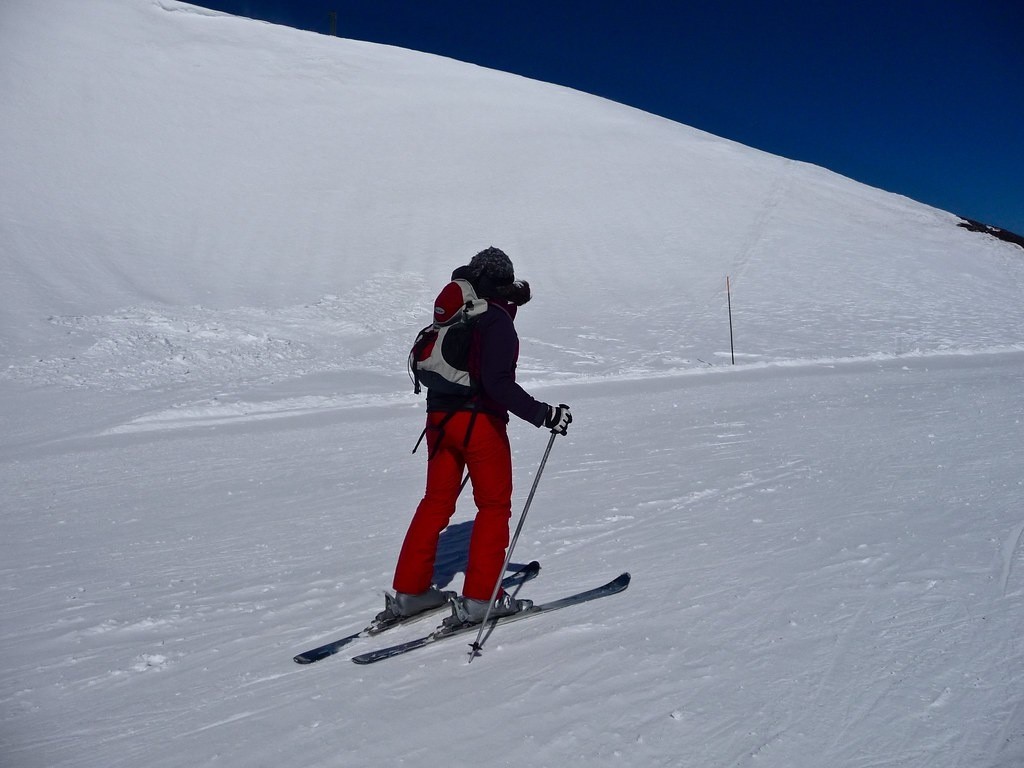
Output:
[542,405,573,437]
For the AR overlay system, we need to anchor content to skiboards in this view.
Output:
[294,561,631,680]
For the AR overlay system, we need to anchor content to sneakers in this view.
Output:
[459,597,519,621]
[394,589,448,616]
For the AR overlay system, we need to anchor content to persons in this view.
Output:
[392,244,573,625]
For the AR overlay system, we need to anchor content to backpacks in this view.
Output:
[408,277,520,399]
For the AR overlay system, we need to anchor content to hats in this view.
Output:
[467,246,514,286]
